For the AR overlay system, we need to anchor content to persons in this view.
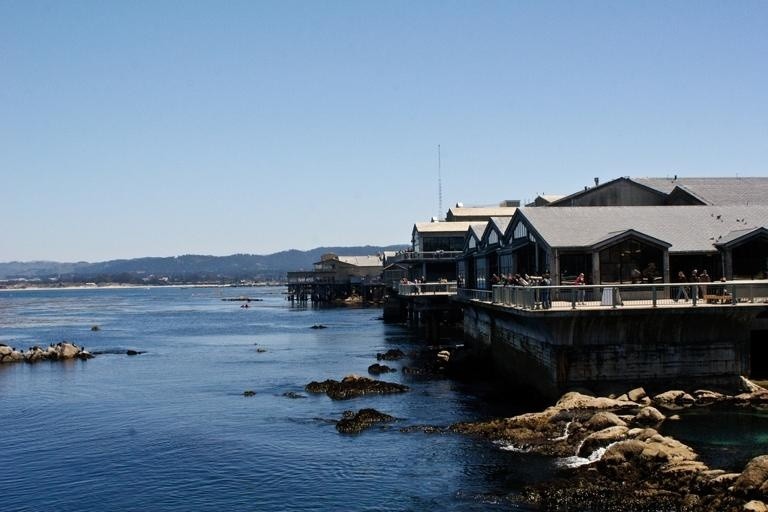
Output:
[575,273,585,305]
[631,266,641,284]
[489,272,553,309]
[689,269,700,300]
[400,273,464,295]
[674,271,688,302]
[698,269,711,298]
